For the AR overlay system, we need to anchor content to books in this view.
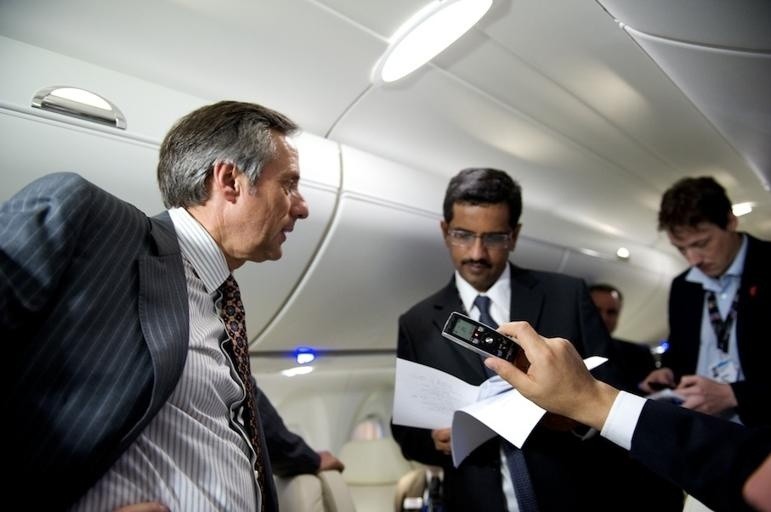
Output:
[480,377,515,400]
[645,387,689,407]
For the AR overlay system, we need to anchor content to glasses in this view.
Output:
[446,224,513,249]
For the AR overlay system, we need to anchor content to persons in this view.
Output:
[388,163,617,512]
[393,461,447,512]
[473,319,770,512]
[637,168,770,435]
[585,282,689,512]
[1,97,346,512]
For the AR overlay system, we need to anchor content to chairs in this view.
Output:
[273,467,355,512]
[338,437,425,512]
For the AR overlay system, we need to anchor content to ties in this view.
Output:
[704,286,742,354]
[472,293,540,511]
[220,276,279,510]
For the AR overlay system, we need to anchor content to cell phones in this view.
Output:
[647,381,676,390]
[441,311,525,365]
